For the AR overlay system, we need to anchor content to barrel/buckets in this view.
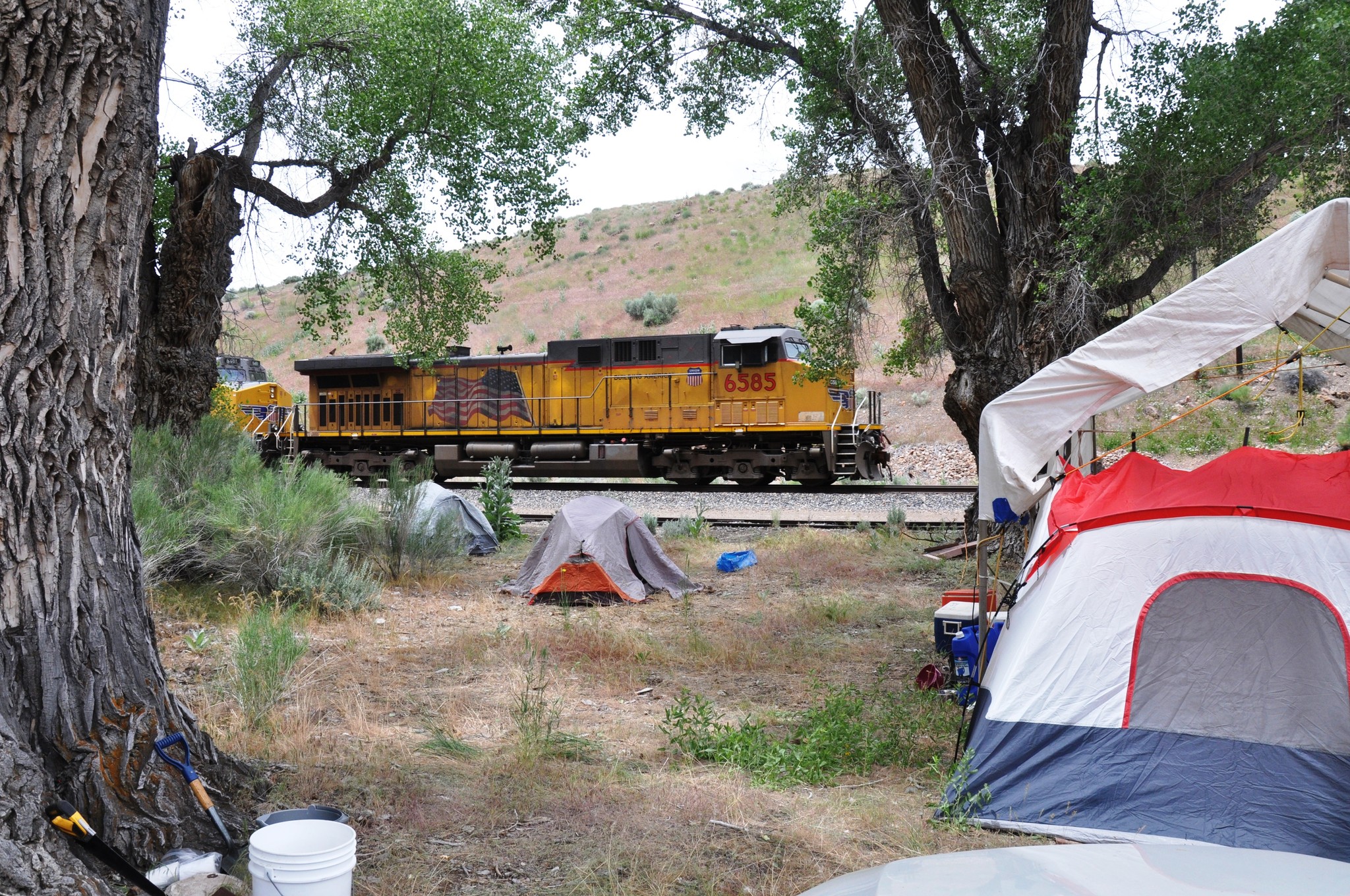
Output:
[247,819,356,896]
[255,804,349,828]
[952,622,1004,706]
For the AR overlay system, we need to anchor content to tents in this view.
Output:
[929,444,1349,865]
[495,495,704,606]
[972,198,1349,687]
[386,482,502,557]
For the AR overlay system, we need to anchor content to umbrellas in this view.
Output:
[795,844,1350,896]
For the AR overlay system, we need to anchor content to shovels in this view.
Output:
[154,732,254,891]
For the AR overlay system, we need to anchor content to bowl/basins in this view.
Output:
[931,690,957,702]
[916,664,943,692]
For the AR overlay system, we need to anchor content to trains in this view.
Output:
[210,353,296,461]
[287,322,892,492]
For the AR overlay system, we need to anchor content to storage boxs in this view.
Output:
[943,589,997,620]
[988,610,1010,633]
[934,601,982,652]
